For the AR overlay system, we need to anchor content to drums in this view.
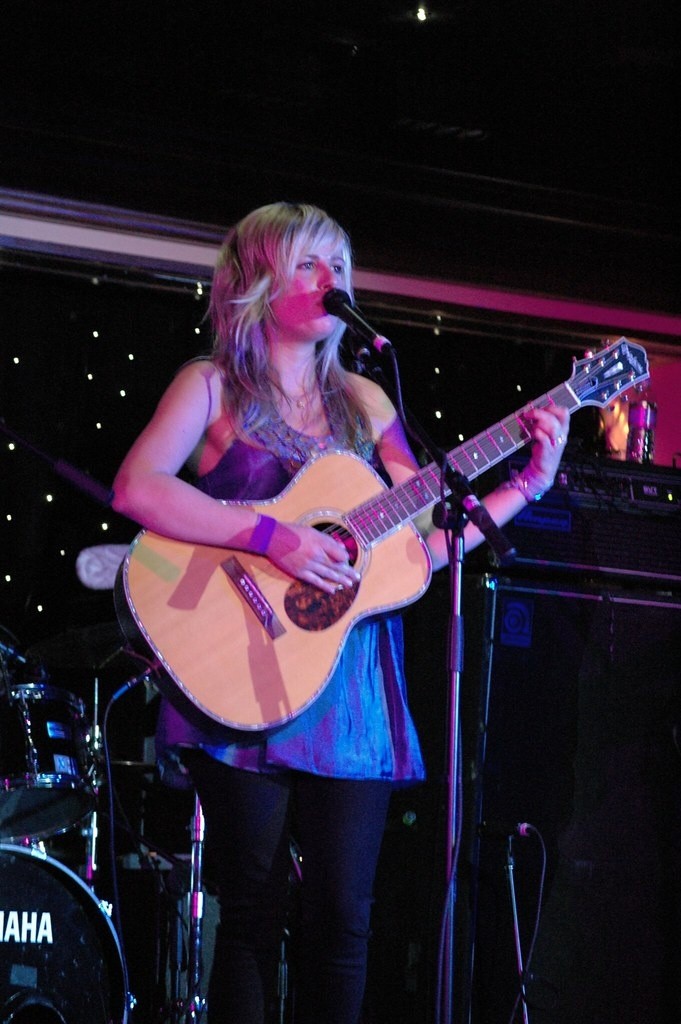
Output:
[0,683,111,841]
[0,844,139,1024]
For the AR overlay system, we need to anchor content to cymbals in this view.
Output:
[106,757,160,773]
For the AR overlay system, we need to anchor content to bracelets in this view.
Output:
[507,463,554,503]
[244,514,277,556]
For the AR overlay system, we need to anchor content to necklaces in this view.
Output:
[292,396,309,409]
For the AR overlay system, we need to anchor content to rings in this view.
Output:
[552,434,567,448]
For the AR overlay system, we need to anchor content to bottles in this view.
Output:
[577,348,657,463]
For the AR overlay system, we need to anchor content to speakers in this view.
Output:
[401,569,681,1024]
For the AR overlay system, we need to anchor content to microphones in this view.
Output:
[476,820,532,841]
[343,329,371,363]
[322,287,391,354]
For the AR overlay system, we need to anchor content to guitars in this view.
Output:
[119,334,653,745]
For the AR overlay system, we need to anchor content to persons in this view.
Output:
[108,202,570,1024]
